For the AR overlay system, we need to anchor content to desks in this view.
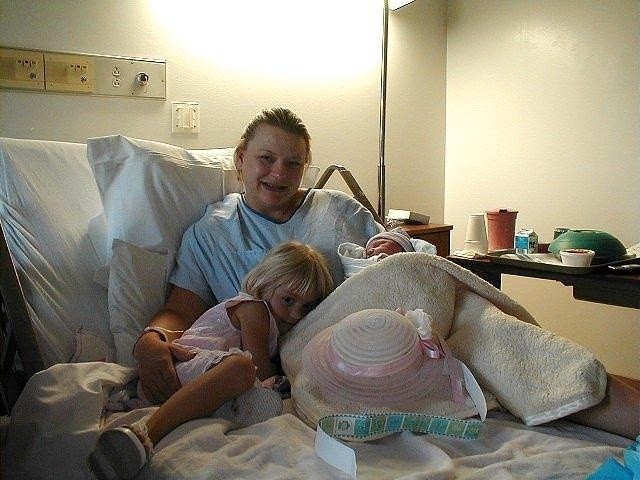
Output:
[447,248,639,310]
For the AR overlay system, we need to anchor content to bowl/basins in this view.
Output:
[559,246,596,267]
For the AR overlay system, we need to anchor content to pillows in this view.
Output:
[87,137,244,366]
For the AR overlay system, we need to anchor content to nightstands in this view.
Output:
[383,221,452,263]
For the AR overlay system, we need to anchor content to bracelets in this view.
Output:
[132,326,170,358]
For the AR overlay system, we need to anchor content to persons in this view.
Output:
[130,107,385,407]
[89,241,337,479]
[363,226,415,259]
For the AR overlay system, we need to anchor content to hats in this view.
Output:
[364,231,412,252]
[303,308,445,407]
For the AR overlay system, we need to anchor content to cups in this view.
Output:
[463,214,489,257]
[485,207,520,253]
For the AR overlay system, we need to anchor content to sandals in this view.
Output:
[88,424,153,480]
[105,379,152,412]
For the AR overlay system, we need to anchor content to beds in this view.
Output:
[2,139,639,479]
